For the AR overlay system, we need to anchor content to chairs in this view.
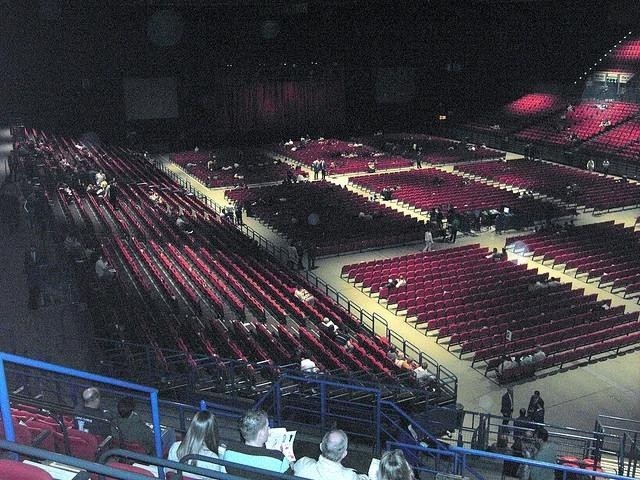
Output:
[14,128,639,400]
[0,401,198,480]
[556,455,607,480]
